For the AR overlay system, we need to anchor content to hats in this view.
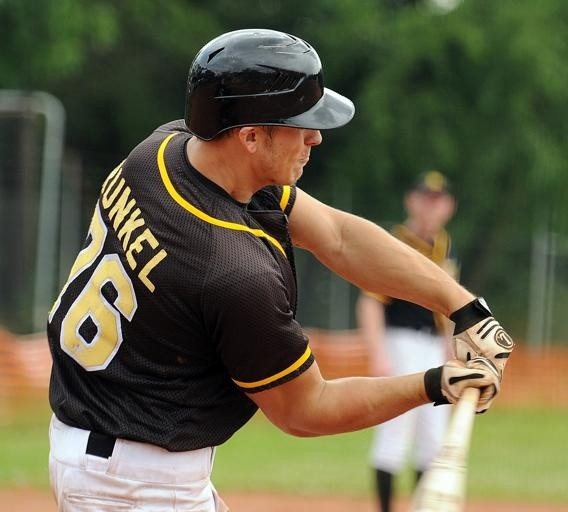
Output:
[408,169,453,195]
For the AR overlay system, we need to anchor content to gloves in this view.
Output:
[422,356,502,414]
[448,296,516,375]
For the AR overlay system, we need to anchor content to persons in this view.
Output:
[43,27,514,512]
[354,168,464,511]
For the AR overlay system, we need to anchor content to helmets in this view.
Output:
[183,28,356,143]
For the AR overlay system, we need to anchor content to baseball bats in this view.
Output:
[409,387,481,507]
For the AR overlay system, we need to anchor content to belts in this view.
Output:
[414,324,440,337]
